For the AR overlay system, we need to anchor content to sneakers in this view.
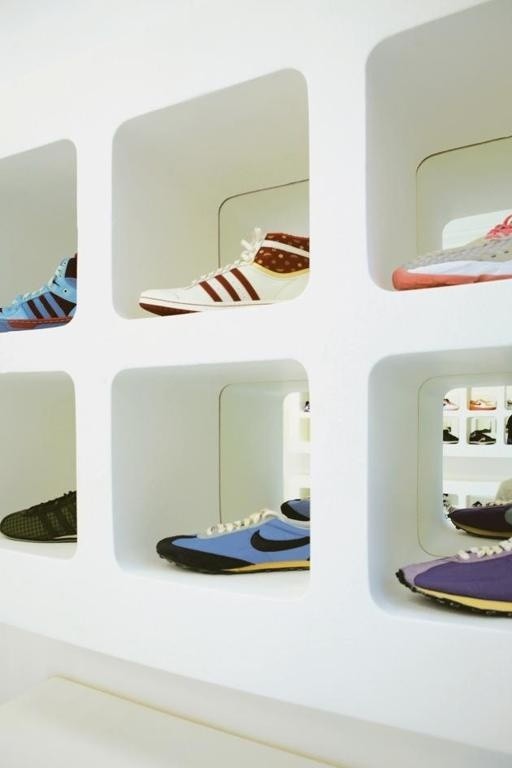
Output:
[446,503,512,539]
[443,429,459,443]
[0,490,77,542]
[469,430,496,445]
[140,233,309,315]
[280,499,309,521]
[469,399,496,409]
[1,254,77,331]
[392,214,512,290]
[396,537,512,616]
[443,398,460,410]
[156,499,310,575]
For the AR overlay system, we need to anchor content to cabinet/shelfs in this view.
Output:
[0,0,511,752]
